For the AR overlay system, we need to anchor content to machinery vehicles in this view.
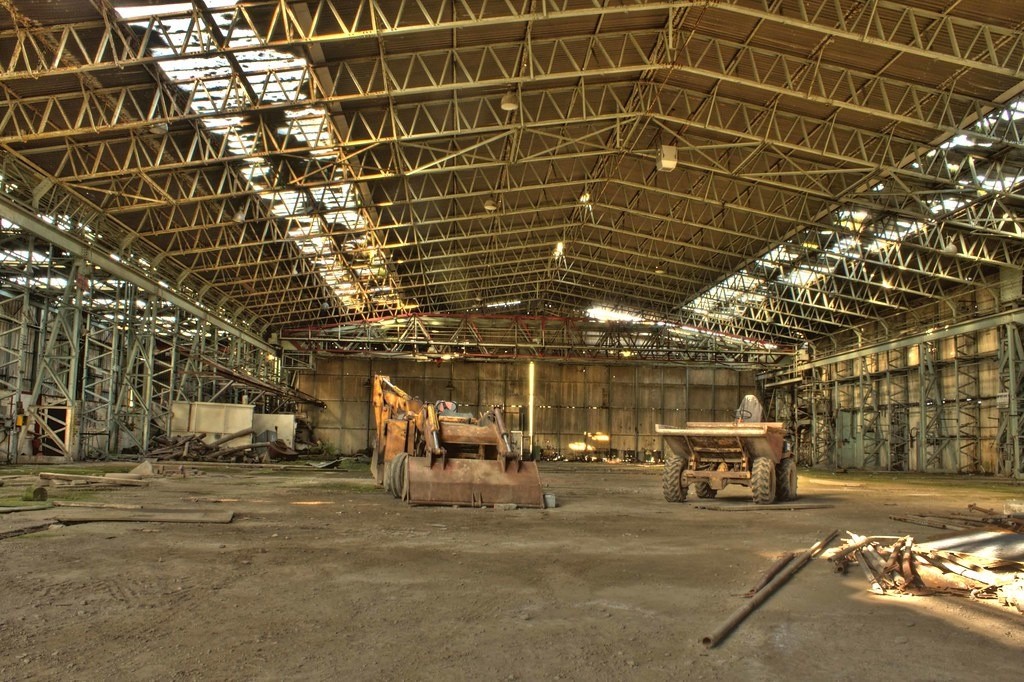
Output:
[371,375,548,510]
[653,394,798,505]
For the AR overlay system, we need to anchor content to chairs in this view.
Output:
[436,400,458,413]
[735,395,763,422]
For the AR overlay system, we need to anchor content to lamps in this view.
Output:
[501,89,519,111]
[654,262,667,274]
[446,362,456,391]
[232,206,246,224]
[363,360,373,389]
[148,104,168,135]
[484,192,498,210]
[654,135,679,173]
[944,235,957,256]
